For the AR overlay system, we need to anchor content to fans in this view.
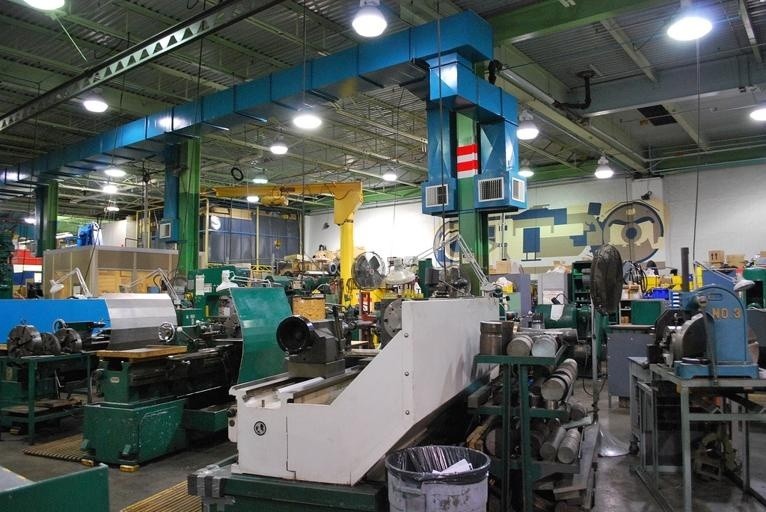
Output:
[590,243,628,457]
[350,250,386,291]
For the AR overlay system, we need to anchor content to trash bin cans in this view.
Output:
[385,446,490,512]
[604,326,650,407]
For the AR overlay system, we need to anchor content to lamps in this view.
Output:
[350,0,386,37]
[515,108,538,140]
[83,86,109,113]
[593,151,613,179]
[385,232,492,297]
[667,1,711,41]
[215,269,270,292]
[48,268,92,298]
[518,157,533,177]
[692,259,755,292]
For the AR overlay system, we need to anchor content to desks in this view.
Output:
[605,323,655,408]
[625,355,766,512]
